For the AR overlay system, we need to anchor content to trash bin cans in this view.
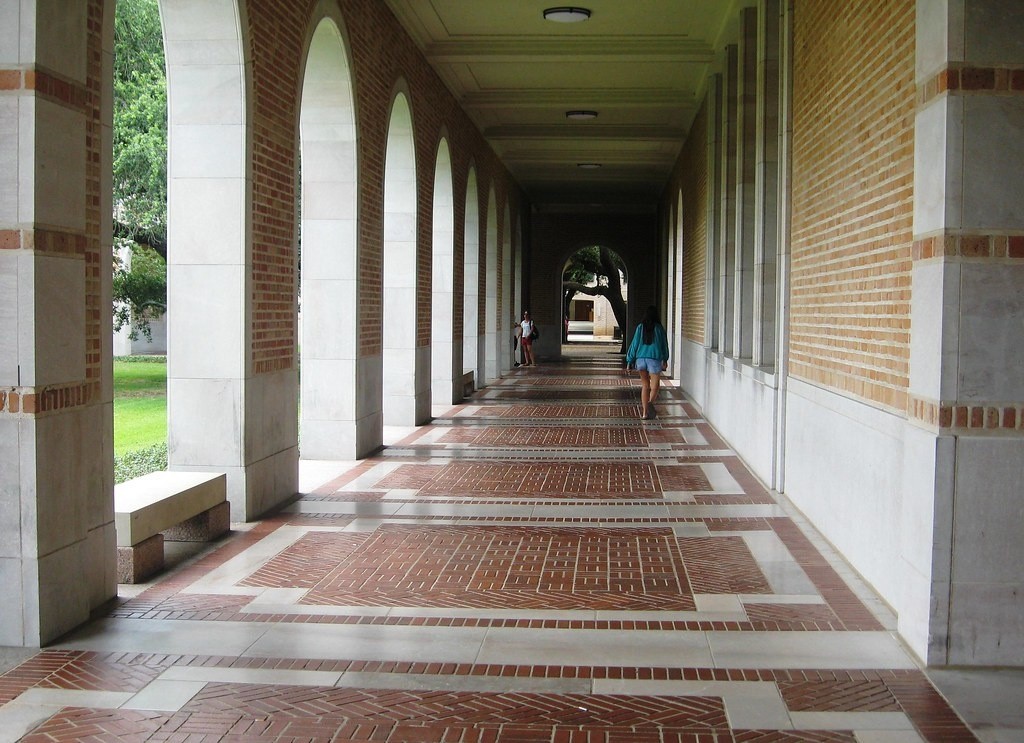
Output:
[614,326,621,339]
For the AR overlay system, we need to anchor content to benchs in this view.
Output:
[115,471,230,585]
[463,368,474,397]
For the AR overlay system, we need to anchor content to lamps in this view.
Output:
[566,110,597,120]
[543,7,590,23]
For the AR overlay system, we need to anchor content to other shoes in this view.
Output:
[647,401,656,419]
[532,362,536,366]
[514,362,520,367]
[640,413,650,420]
[523,363,529,366]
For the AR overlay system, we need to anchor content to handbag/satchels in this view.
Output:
[530,319,540,340]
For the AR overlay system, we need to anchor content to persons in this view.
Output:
[517,310,536,366]
[626,305,669,420]
[514,321,521,367]
[565,312,570,343]
